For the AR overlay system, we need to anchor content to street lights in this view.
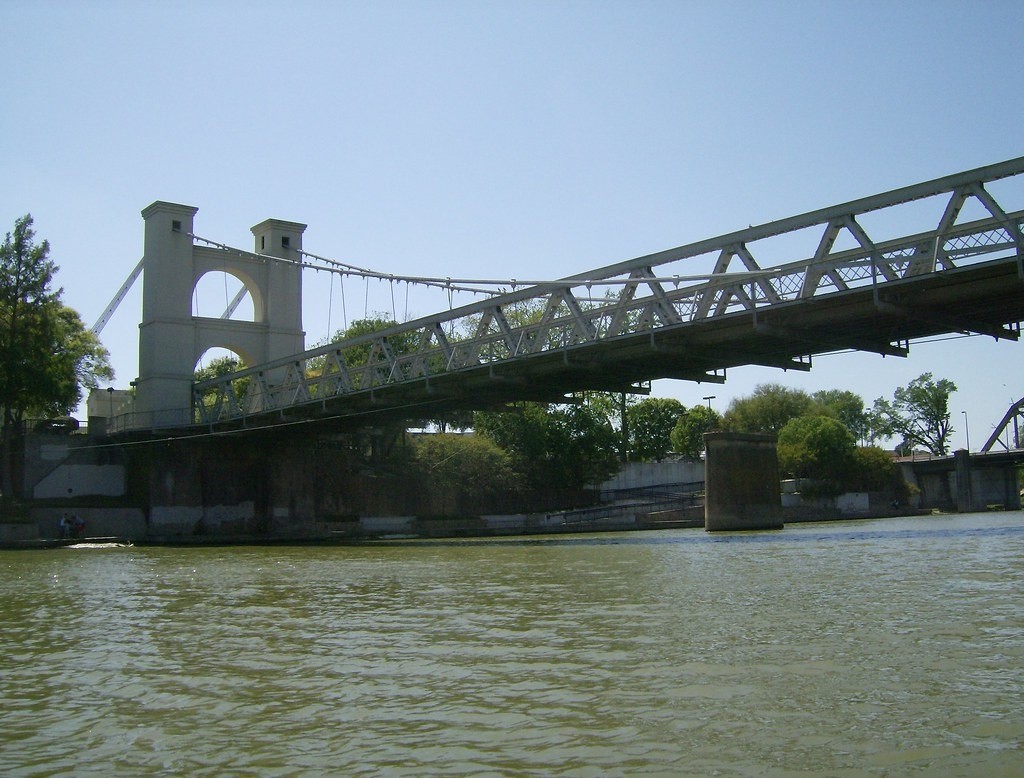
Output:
[962,411,970,451]
[107,387,115,416]
[702,396,715,410]
[866,407,874,446]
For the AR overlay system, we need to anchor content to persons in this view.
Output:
[55,507,84,535]
